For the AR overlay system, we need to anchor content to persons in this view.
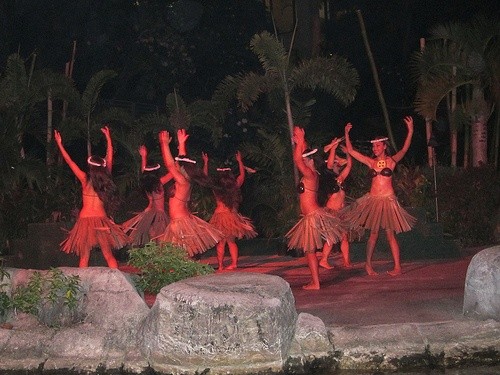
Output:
[159,128,224,258]
[284,126,349,290]
[345,115,418,276]
[202,150,258,273]
[55,126,129,268]
[120,145,174,247]
[318,137,359,269]
